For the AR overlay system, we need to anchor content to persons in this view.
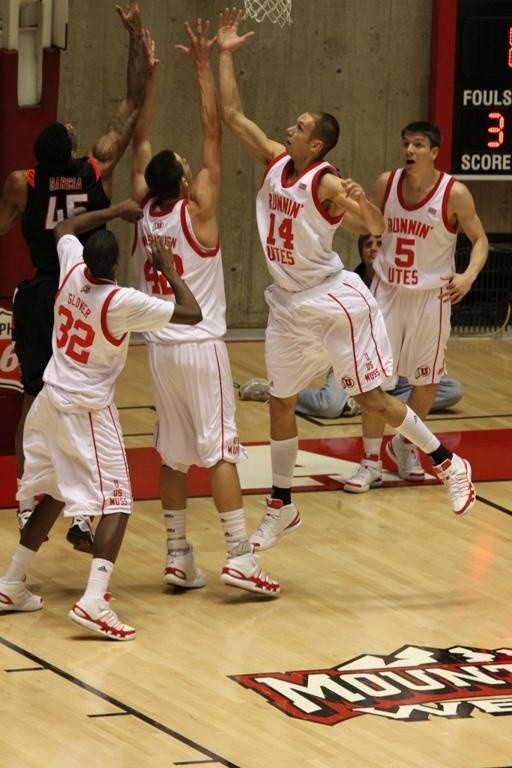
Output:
[237,233,464,420]
[130,16,280,600]
[0,0,153,552]
[340,121,490,493]
[217,6,476,551]
[0,199,203,641]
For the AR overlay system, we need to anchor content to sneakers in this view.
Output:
[342,457,384,493]
[0,576,44,611]
[384,433,424,483]
[431,452,477,516]
[248,495,304,553]
[65,515,96,552]
[68,592,137,641]
[16,510,49,543]
[161,543,208,588]
[220,552,281,598]
[237,376,274,402]
